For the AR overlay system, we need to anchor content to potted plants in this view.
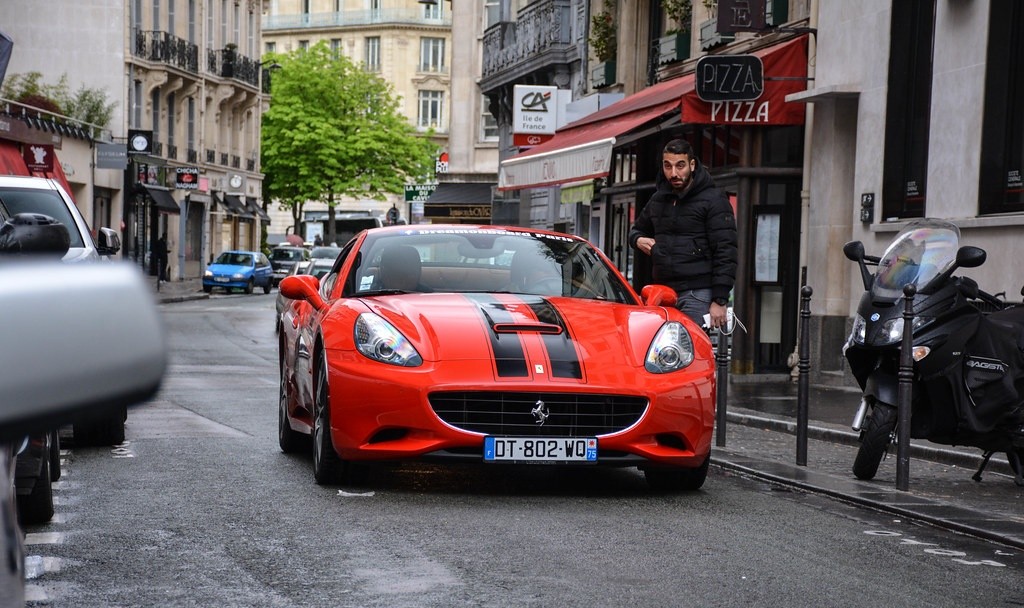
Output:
[699,0,736,51]
[41,93,60,118]
[10,70,43,114]
[24,83,44,117]
[658,0,692,64]
[589,12,615,90]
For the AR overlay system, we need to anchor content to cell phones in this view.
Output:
[702,308,732,329]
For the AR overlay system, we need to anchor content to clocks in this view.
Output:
[230,177,242,188]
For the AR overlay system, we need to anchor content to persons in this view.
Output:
[322,230,330,246]
[156,233,171,284]
[314,235,323,246]
[503,247,602,298]
[627,140,739,338]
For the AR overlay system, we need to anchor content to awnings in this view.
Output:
[424,183,504,218]
[497,36,809,191]
[560,178,602,203]
[145,187,180,216]
[224,196,254,223]
[0,139,77,203]
[246,197,271,226]
[209,193,240,216]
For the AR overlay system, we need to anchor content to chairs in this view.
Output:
[510,249,540,282]
[369,245,434,292]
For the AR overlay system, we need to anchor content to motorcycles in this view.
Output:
[840,217,1024,487]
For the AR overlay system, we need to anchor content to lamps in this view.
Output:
[255,59,282,73]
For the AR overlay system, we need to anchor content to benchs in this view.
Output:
[366,267,511,291]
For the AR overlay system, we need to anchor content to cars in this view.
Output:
[0,176,171,608]
[272,223,716,493]
[202,251,273,294]
